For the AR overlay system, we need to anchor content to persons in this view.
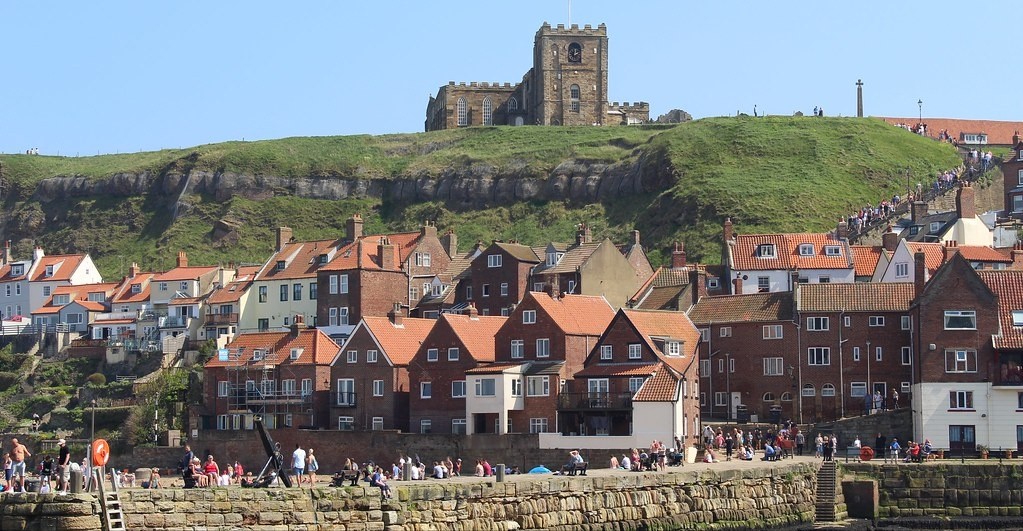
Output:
[754,104,758,117]
[10,438,32,492]
[834,118,992,241]
[630,425,935,469]
[619,453,631,470]
[813,106,818,116]
[892,388,900,410]
[56,438,72,496]
[863,391,872,415]
[305,448,318,489]
[557,451,579,476]
[35,455,522,499]
[572,449,584,475]
[1,452,14,492]
[609,454,620,469]
[291,443,306,486]
[182,444,195,489]
[874,390,883,412]
[818,107,824,117]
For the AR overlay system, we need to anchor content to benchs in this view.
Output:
[330,469,361,488]
[765,449,781,461]
[183,470,197,489]
[566,461,589,476]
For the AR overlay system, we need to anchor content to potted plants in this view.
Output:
[977,445,990,459]
[937,447,945,459]
[1005,448,1013,459]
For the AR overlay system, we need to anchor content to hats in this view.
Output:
[57,438,66,445]
